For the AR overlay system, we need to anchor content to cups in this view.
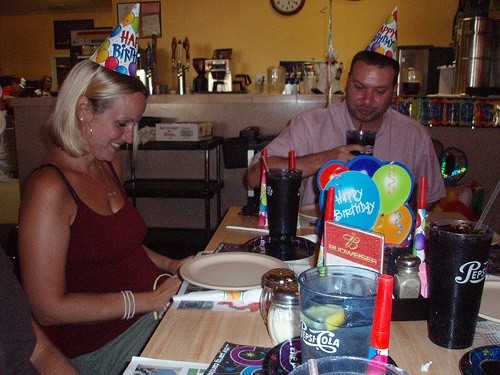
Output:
[287,265,409,375]
[266,65,286,95]
[346,130,377,157]
[401,68,423,95]
[265,167,303,244]
[427,219,494,349]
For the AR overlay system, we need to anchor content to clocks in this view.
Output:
[270,0,305,15]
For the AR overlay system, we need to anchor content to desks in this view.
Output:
[140,206,500,375]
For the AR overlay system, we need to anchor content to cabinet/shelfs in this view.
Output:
[119,124,224,243]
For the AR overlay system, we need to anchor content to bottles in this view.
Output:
[394,254,422,299]
[259,268,300,346]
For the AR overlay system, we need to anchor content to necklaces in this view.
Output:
[86,159,117,198]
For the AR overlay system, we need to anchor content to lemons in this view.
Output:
[299,303,346,333]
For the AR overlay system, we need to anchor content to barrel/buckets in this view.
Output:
[452,16,500,96]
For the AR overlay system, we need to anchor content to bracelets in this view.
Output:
[120,290,136,319]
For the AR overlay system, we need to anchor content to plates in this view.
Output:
[459,345,500,375]
[179,252,288,291]
[243,236,316,263]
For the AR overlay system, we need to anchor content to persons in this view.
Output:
[18,3,195,375]
[245,5,447,222]
[0,247,81,375]
